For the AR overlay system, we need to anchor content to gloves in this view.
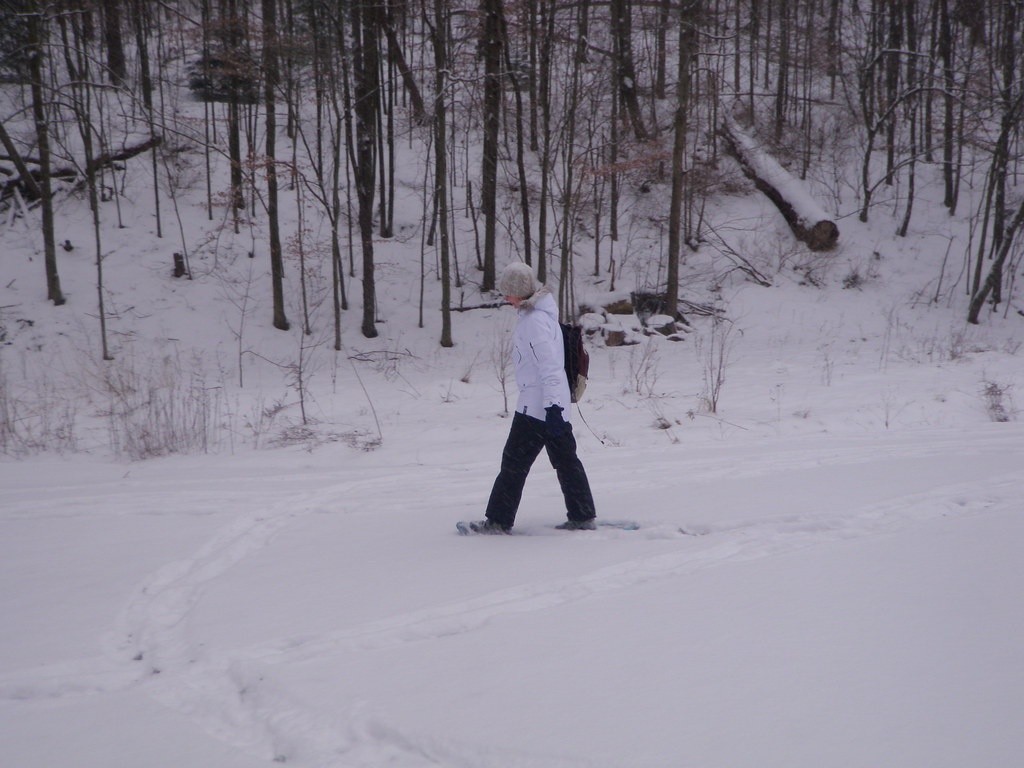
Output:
[543,404,572,443]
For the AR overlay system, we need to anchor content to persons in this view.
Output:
[456,262,601,535]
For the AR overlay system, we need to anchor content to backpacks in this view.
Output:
[558,321,590,403]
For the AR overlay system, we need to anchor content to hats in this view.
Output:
[499,262,541,298]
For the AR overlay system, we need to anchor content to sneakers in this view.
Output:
[456,518,512,537]
[555,517,597,531]
